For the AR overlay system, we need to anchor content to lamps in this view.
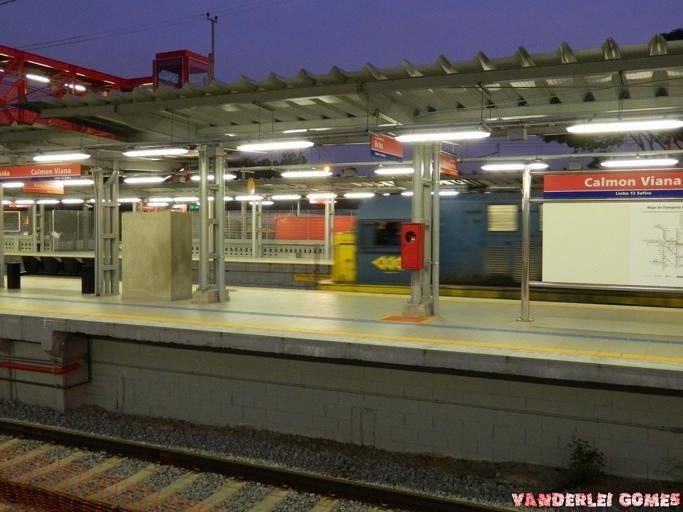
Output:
[2,110,682,190]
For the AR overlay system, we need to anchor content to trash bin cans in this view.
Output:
[6,262,18,289]
[81,262,93,293]
